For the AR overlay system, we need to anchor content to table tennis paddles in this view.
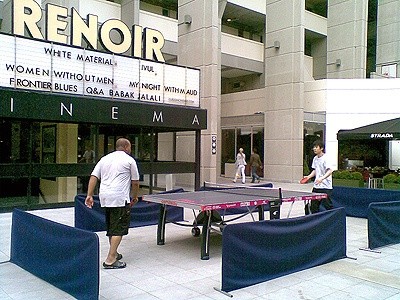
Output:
[300,177,309,184]
[129,199,137,207]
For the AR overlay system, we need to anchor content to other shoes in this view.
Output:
[250,180,255,183]
[242,181,245,184]
[232,180,236,183]
[258,178,261,183]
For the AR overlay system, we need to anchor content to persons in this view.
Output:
[232,148,247,184]
[85,138,139,269]
[249,148,262,183]
[303,140,334,214]
[79,141,96,163]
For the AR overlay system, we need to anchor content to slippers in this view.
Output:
[103,253,126,269]
